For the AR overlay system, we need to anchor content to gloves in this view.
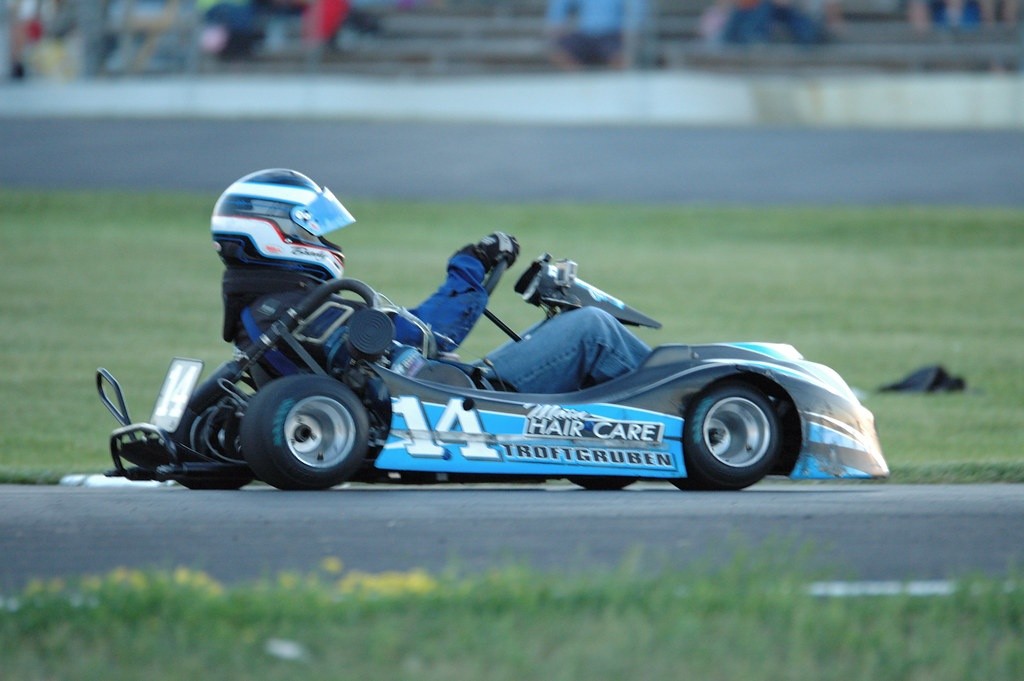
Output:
[478,231,520,269]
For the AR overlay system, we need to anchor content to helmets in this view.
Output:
[210,167,357,283]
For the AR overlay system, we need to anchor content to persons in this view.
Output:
[210,167,652,393]
[0,0,1023,79]
